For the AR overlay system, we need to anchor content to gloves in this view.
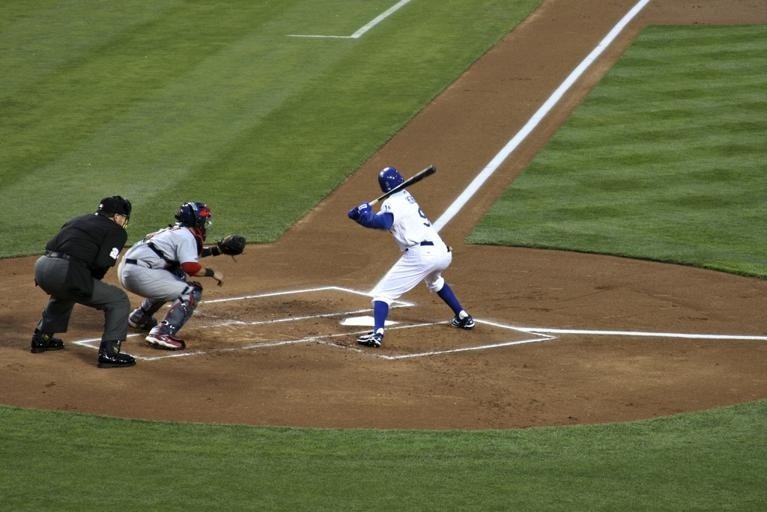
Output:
[348,207,360,219]
[358,203,371,214]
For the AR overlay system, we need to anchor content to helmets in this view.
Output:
[176,201,213,241]
[99,196,131,229]
[378,167,404,192]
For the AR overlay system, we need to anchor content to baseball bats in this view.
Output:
[368,165,435,210]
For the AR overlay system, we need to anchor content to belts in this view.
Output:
[44,251,69,259]
[126,259,137,264]
[405,241,433,250]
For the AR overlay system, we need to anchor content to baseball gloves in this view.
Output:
[216,235,245,255]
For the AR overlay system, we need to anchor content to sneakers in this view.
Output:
[357,333,383,347]
[144,334,185,350]
[128,310,158,329]
[451,315,475,328]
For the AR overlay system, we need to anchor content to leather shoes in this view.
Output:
[98,352,136,367]
[31,336,63,353]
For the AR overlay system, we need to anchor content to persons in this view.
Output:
[118,202,225,350]
[348,166,475,347]
[31,196,136,368]
